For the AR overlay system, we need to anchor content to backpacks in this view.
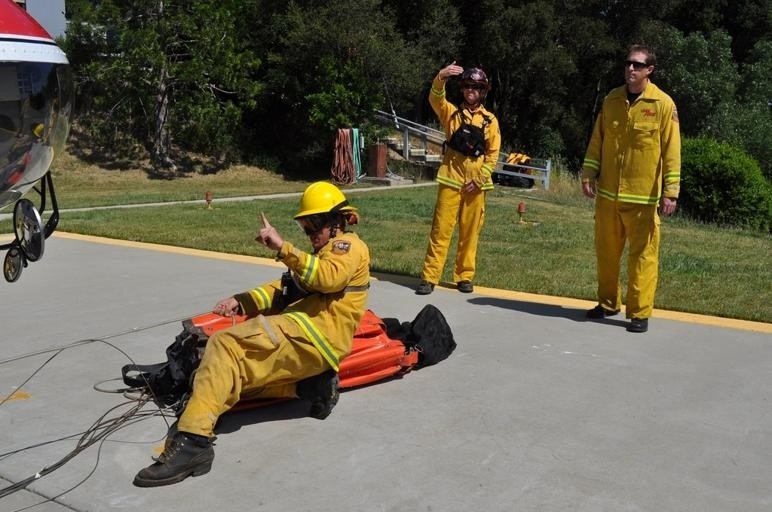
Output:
[446,108,492,157]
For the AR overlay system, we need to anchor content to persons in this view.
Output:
[581,42,683,332]
[415,60,503,295]
[129,179,370,489]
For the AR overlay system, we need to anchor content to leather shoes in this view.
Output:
[133,432,215,486]
[627,318,648,332]
[416,280,434,294]
[296,369,339,420]
[586,306,621,318]
[457,280,473,292]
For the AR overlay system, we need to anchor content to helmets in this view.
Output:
[293,182,358,220]
[461,67,488,85]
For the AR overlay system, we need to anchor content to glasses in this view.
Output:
[624,60,647,68]
[464,83,480,89]
[304,221,325,235]
